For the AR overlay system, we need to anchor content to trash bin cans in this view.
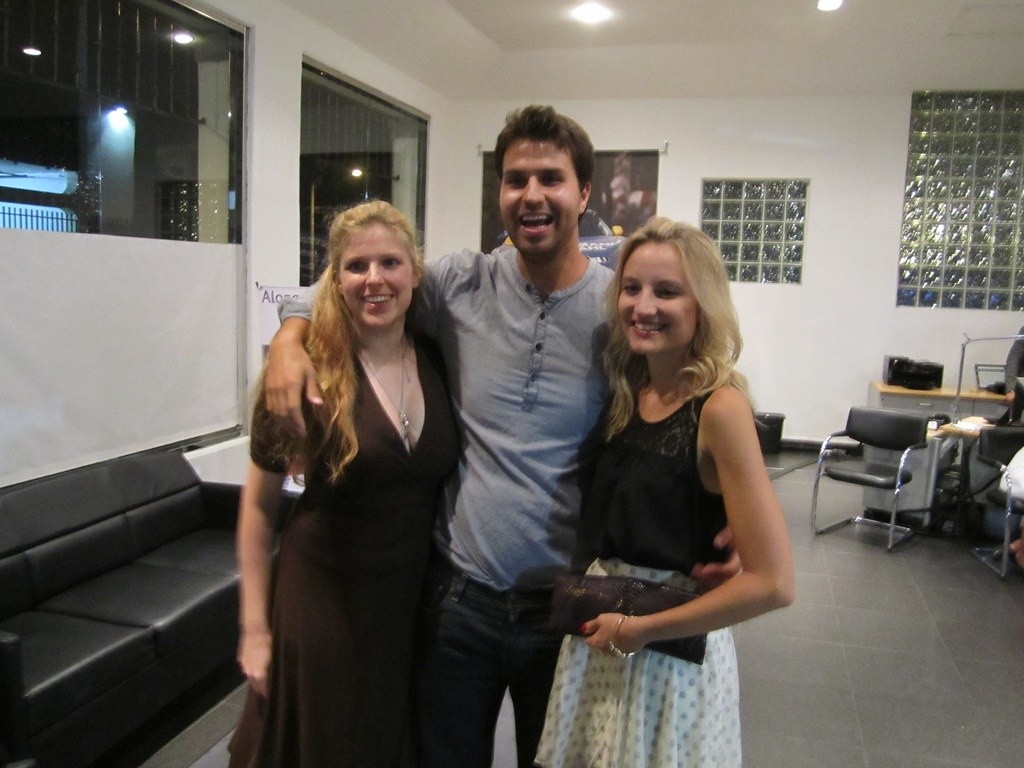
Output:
[754,412,785,453]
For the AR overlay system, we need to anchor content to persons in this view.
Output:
[225,199,468,768]
[261,106,747,767]
[536,216,796,768]
[1003,325,1024,572]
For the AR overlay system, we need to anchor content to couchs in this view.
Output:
[0,449,304,768]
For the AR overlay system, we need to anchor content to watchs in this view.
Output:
[1010,389,1015,392]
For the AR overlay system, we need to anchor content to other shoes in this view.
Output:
[992,542,1024,576]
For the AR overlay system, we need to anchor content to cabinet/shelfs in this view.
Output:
[861,382,1013,525]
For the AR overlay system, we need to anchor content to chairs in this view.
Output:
[970,426,1024,578]
[812,406,929,552]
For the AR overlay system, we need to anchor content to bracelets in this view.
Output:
[608,614,640,659]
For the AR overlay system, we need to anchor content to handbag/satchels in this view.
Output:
[551,574,707,665]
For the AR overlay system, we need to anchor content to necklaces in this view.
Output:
[358,339,411,441]
